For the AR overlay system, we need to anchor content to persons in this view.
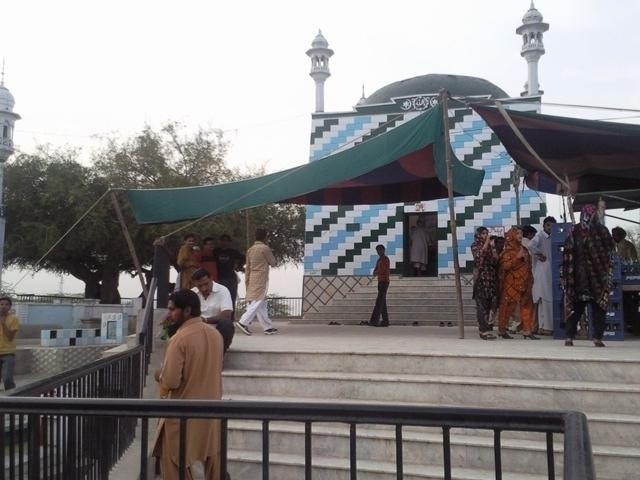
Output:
[1,297,20,390]
[368,244,390,327]
[157,266,234,373]
[470,215,558,340]
[410,218,432,278]
[558,203,616,347]
[191,237,218,282]
[236,229,281,335]
[176,233,199,290]
[213,233,246,322]
[612,226,637,261]
[151,289,224,480]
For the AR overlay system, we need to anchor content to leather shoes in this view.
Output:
[479,332,498,340]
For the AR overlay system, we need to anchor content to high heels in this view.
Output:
[522,333,541,340]
[497,331,514,340]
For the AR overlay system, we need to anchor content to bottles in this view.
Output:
[622,259,639,280]
[603,303,620,334]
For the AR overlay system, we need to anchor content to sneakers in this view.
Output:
[263,328,279,334]
[235,320,253,335]
